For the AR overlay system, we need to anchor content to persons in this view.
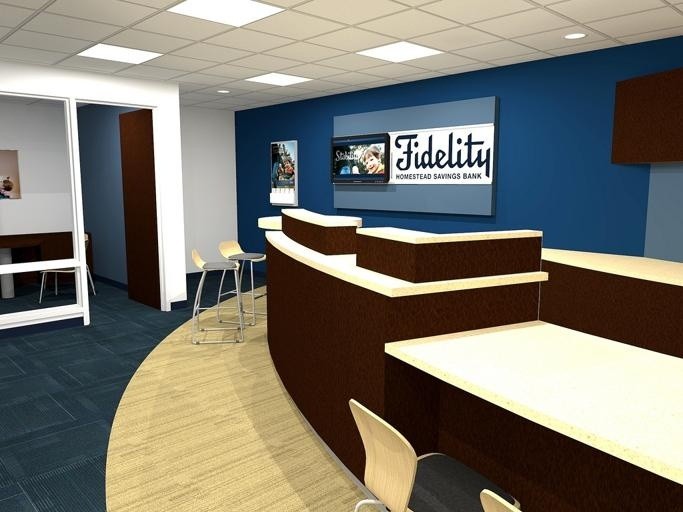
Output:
[363,145,384,174]
[272,159,294,188]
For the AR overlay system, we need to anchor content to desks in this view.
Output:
[0,231,94,299]
[384,316,682,485]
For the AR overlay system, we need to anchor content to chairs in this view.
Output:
[190,250,245,344]
[347,399,521,512]
[217,241,267,326]
[479,489,523,511]
[38,233,96,304]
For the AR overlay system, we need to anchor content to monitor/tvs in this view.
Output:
[333,134,388,183]
